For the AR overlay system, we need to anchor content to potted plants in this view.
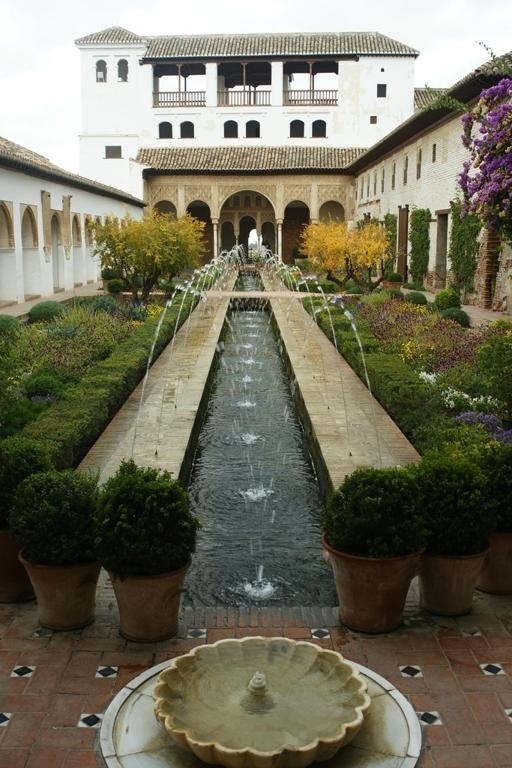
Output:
[321,468,429,634]
[14,468,105,634]
[98,459,203,639]
[100,265,124,302]
[417,458,499,620]
[382,271,403,300]
